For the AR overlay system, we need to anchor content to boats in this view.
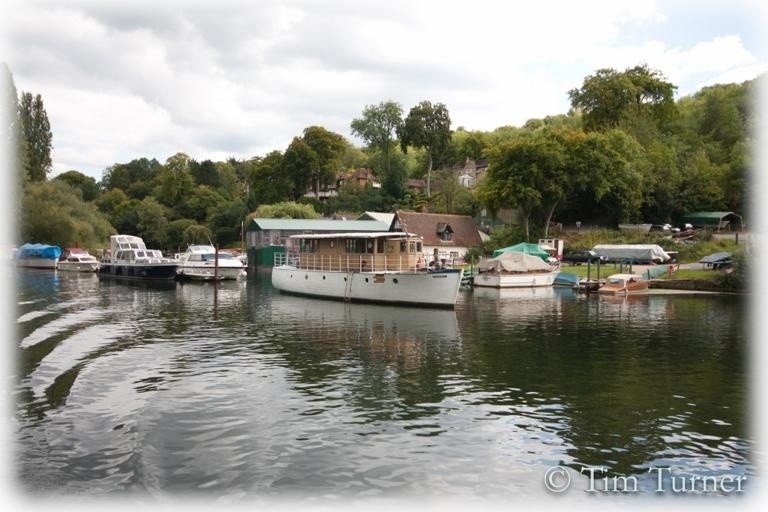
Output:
[462,251,561,288]
[597,274,651,295]
[271,231,465,305]
[552,271,580,287]
[0,234,247,282]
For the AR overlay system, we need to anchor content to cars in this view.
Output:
[544,249,563,263]
[565,250,608,265]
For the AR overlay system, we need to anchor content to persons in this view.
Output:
[433,248,440,269]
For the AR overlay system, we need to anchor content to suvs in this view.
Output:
[673,222,693,232]
[646,223,681,240]
